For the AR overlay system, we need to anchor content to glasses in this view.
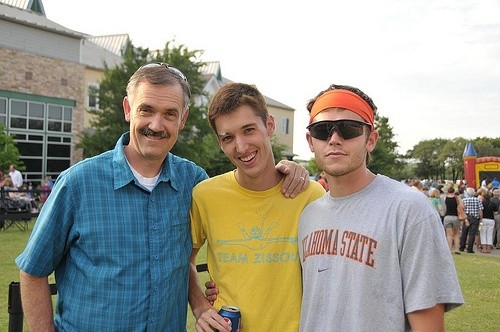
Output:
[305,119,370,140]
[138,62,187,82]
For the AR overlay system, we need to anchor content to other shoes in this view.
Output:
[454,251,461,254]
[480,250,490,253]
[467,251,475,253]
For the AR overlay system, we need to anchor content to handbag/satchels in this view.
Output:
[455,195,467,221]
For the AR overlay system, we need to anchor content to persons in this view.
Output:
[0,164,54,211]
[187,83,326,332]
[205,84,463,332]
[309,171,330,193]
[14,63,310,332]
[405,179,500,255]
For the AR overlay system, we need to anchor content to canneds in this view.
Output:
[218,305,241,332]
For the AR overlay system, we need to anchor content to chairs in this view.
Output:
[0,199,31,232]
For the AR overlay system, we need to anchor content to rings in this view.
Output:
[300,177,305,180]
